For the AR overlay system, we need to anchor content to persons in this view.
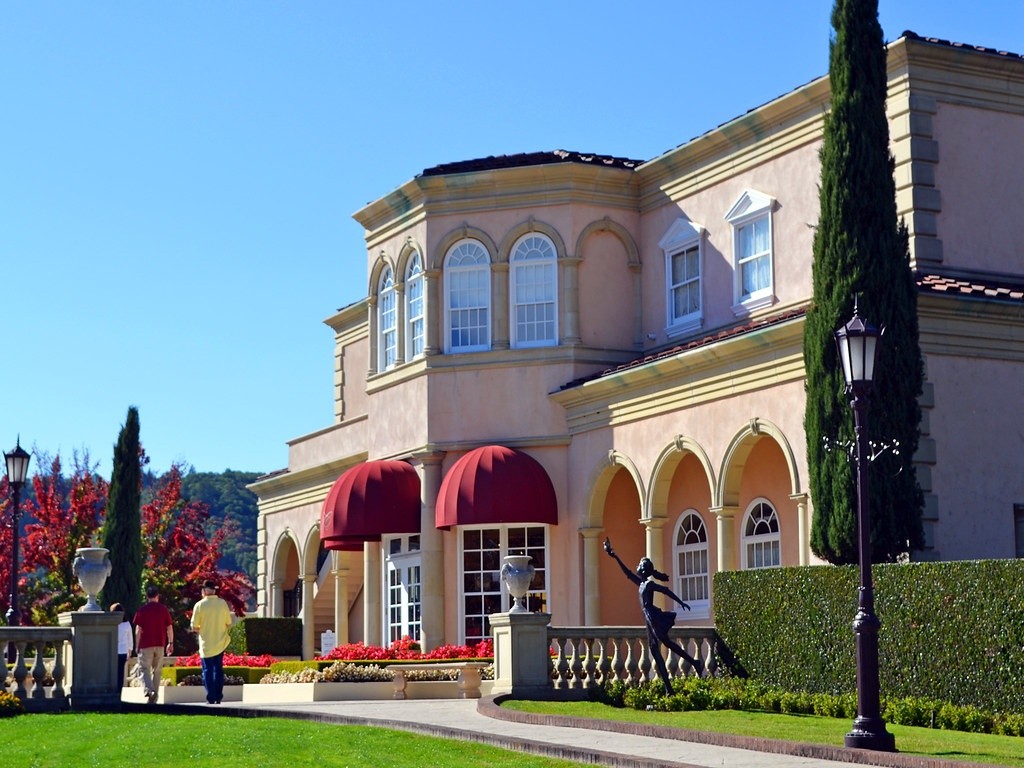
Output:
[132,586,174,703]
[603,536,703,696]
[110,602,134,699]
[190,580,233,704]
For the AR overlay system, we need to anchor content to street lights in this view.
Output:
[2,432,32,663]
[833,295,898,753]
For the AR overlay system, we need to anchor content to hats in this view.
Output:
[199,580,218,589]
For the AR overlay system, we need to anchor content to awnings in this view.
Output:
[434,444,558,531]
[319,460,422,551]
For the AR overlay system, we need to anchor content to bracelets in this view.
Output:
[168,642,173,644]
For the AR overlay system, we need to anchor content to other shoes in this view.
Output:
[147,691,157,704]
